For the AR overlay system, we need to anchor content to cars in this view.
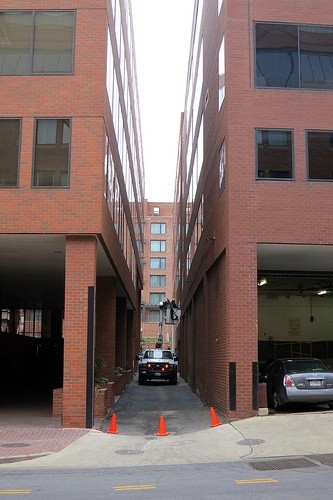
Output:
[263,358,333,412]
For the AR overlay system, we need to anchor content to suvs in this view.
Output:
[136,349,178,385]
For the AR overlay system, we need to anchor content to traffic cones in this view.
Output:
[209,406,223,427]
[107,413,119,434]
[157,415,169,436]
